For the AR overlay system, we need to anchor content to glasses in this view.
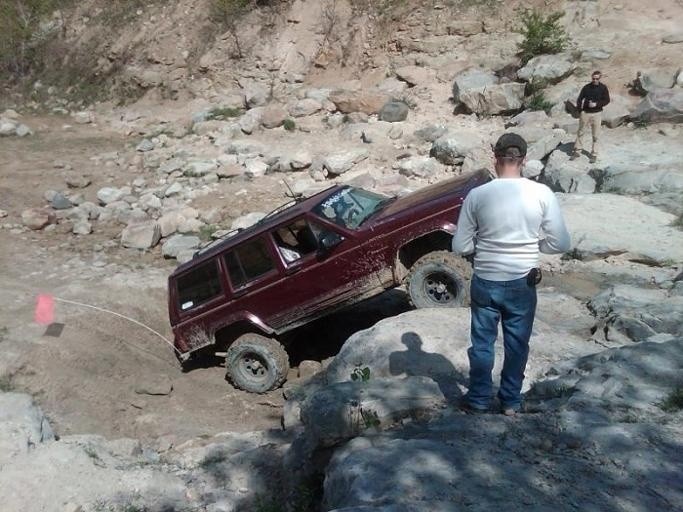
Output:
[592,78,599,81]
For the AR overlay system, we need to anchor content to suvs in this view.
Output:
[165,166,496,394]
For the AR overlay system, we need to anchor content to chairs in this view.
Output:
[261,224,335,267]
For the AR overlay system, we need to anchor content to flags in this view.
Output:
[34,293,56,323]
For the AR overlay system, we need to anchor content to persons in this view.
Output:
[446,131,571,419]
[570,70,610,164]
[295,205,347,254]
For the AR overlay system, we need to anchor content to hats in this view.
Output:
[491,132,527,159]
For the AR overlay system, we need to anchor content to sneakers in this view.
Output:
[459,393,487,415]
[589,154,596,163]
[568,152,580,161]
[502,394,526,415]
